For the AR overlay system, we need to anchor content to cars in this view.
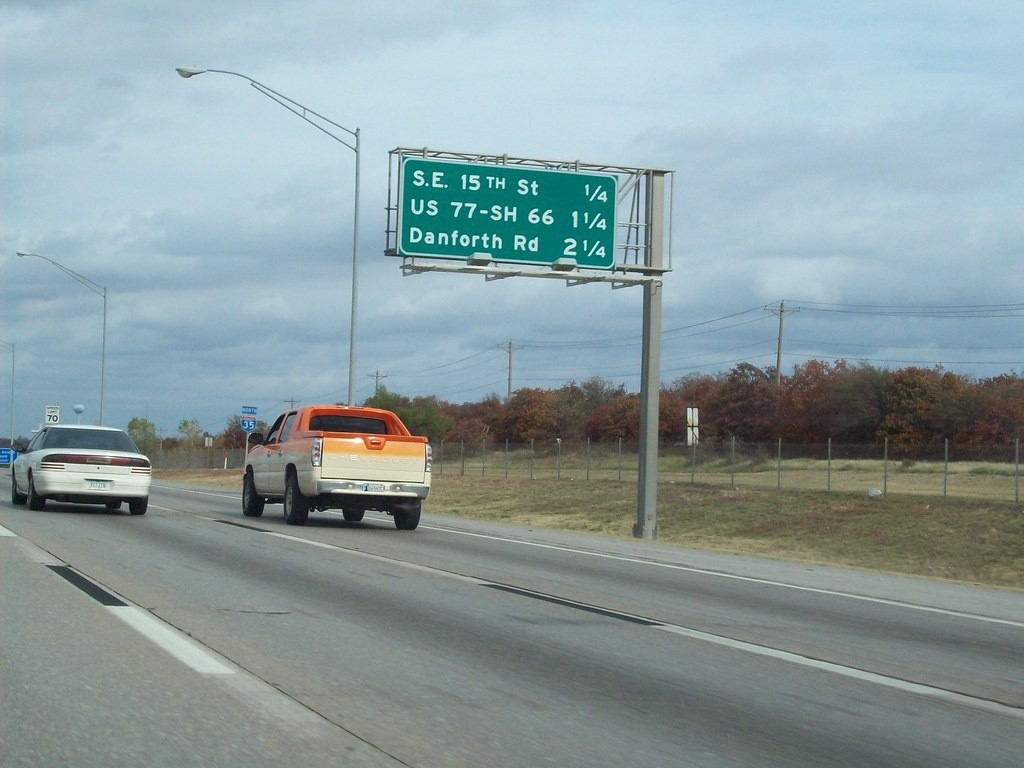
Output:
[11,425,151,516]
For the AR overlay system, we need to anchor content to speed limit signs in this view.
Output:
[45,406,60,423]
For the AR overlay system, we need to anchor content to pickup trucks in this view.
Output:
[241,404,433,531]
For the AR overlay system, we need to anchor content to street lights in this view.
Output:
[173,65,362,409]
[15,248,108,425]
[73,403,85,424]
